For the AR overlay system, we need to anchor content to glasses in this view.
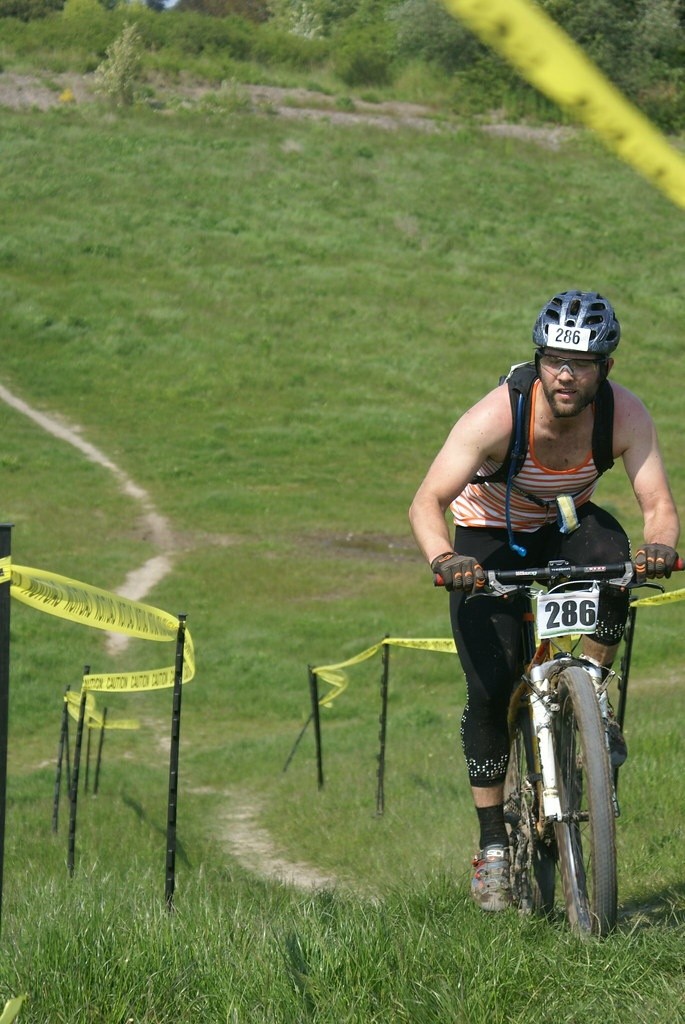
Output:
[535,350,605,378]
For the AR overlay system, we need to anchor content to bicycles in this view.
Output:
[434,553,684,938]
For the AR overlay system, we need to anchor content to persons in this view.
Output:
[406,291,680,912]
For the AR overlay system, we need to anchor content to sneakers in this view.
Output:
[472,844,511,912]
[605,723,626,769]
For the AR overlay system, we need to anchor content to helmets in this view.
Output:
[532,291,621,353]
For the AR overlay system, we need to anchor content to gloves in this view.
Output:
[633,543,678,585]
[429,552,486,594]
